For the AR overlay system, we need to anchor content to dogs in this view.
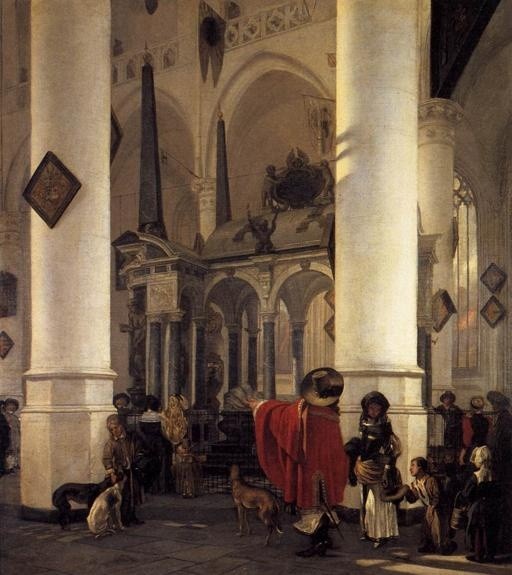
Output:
[228,460,285,548]
[51,472,128,539]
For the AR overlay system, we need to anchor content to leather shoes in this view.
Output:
[295,538,333,557]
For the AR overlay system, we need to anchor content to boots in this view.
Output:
[120,504,144,527]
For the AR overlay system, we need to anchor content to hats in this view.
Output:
[486,391,510,410]
[301,367,344,407]
[440,391,456,402]
[3,398,19,411]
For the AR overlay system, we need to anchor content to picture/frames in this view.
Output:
[22,150,82,230]
[323,286,335,342]
[431,263,507,333]
[0,331,15,360]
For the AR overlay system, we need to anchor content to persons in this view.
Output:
[344,385,512,564]
[122,299,146,379]
[246,367,350,560]
[101,393,168,526]
[0,398,21,478]
[267,165,279,206]
[313,158,334,196]
[253,220,278,255]
